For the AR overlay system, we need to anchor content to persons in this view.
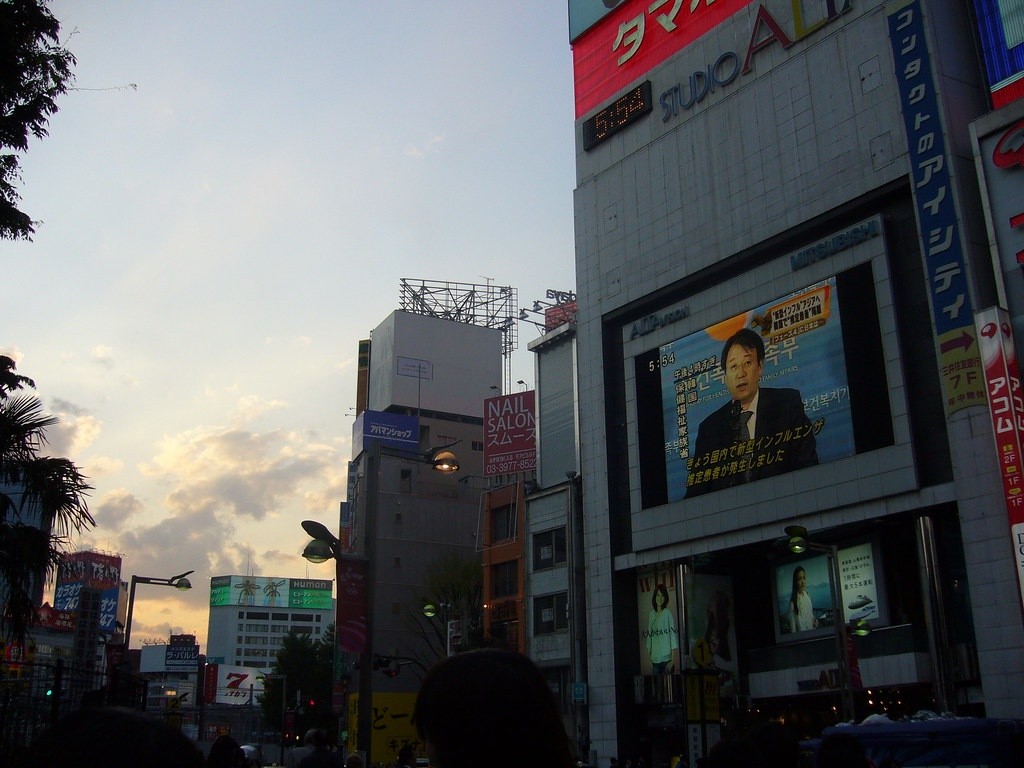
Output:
[647,585,678,702]
[788,566,819,633]
[286,730,363,768]
[703,710,799,768]
[683,328,819,500]
[397,745,417,768]
[818,732,867,768]
[414,649,578,768]
[21,707,260,768]
[609,757,687,768]
[704,576,734,661]
[880,757,899,768]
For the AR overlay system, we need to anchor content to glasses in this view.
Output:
[726,360,757,372]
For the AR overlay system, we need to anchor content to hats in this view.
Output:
[240,745,259,760]
[304,729,316,739]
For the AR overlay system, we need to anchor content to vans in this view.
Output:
[809,712,1024,768]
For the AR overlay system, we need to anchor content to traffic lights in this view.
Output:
[301,699,320,707]
[383,657,401,677]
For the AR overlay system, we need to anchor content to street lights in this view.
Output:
[784,522,858,724]
[420,596,450,658]
[122,570,196,670]
[255,670,288,767]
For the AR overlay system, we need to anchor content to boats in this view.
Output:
[847,593,873,610]
[849,603,876,622]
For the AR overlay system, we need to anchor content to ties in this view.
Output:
[737,411,753,482]
